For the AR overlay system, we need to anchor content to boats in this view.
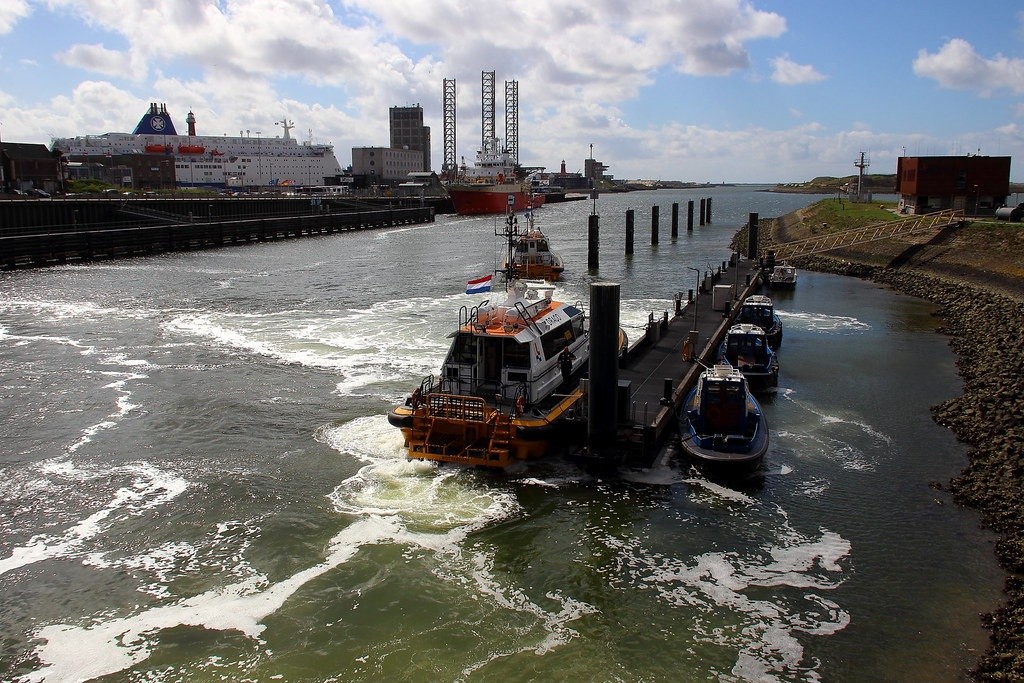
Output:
[178,142,207,154]
[675,363,770,465]
[447,136,546,216]
[716,323,779,388]
[733,294,782,345]
[388,278,631,467]
[769,264,798,290]
[494,192,564,282]
[143,144,174,152]
[209,150,224,155]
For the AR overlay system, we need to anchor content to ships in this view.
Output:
[49,105,344,187]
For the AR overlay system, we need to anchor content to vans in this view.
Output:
[102,189,119,195]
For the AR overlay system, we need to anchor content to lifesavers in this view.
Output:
[517,396,524,414]
[412,388,422,406]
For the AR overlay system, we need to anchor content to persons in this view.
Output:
[557,346,576,386]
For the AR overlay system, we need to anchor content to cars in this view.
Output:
[232,191,288,197]
[8,187,51,198]
[143,191,159,196]
[123,192,138,197]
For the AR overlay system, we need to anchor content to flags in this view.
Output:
[466,274,492,294]
[269,179,279,185]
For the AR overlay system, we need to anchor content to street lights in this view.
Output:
[309,165,312,197]
